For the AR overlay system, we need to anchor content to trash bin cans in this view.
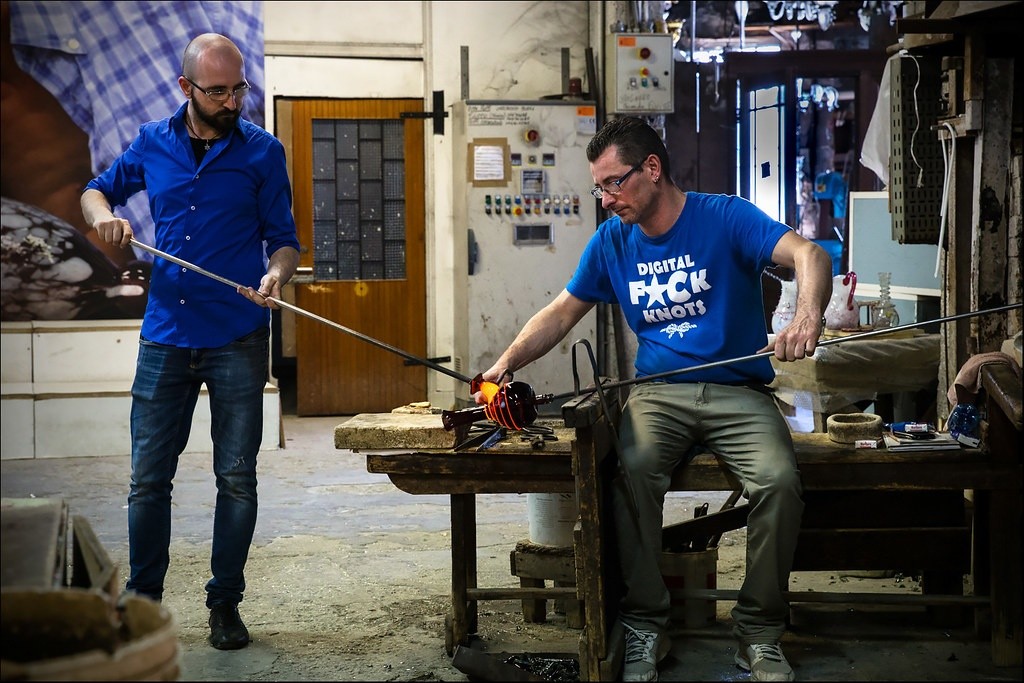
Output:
[0,585,181,683]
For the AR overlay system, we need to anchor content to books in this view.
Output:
[882,431,962,451]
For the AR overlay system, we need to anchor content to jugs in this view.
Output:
[824,271,860,330]
[771,280,798,334]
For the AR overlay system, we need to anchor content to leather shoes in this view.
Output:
[209,608,249,650]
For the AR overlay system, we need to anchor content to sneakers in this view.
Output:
[733,640,795,683]
[618,616,673,683]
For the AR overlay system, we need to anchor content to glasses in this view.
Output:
[182,74,253,102]
[589,157,646,199]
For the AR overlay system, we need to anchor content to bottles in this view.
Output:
[872,271,899,329]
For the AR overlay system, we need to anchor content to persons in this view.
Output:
[80,32,303,651]
[475,116,833,683]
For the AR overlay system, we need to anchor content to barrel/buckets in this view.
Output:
[526,492,576,548]
[659,544,719,628]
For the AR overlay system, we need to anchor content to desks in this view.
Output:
[360,436,985,665]
[764,331,939,432]
